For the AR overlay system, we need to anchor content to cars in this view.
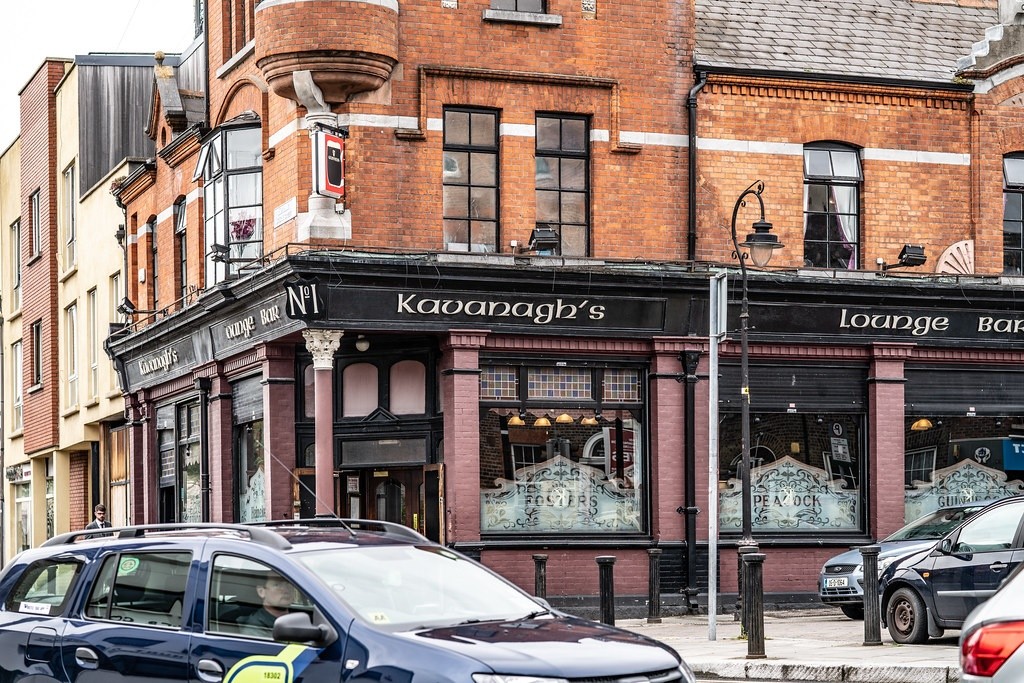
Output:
[816,501,998,621]
[957,557,1024,683]
[877,495,1024,643]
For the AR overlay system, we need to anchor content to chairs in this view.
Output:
[105,574,161,613]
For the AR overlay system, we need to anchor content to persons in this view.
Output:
[85,505,114,539]
[241,577,295,638]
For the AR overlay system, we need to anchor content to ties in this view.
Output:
[101,523,105,537]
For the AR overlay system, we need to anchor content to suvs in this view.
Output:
[0,513,698,683]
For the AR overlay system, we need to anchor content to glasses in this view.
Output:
[96,511,105,515]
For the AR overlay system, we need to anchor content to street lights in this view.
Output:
[732,182,784,627]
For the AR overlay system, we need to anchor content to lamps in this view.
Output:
[519,228,558,253]
[121,296,135,313]
[556,413,574,423]
[211,243,231,264]
[911,418,933,430]
[581,417,599,424]
[534,418,552,426]
[883,244,927,270]
[508,415,526,425]
